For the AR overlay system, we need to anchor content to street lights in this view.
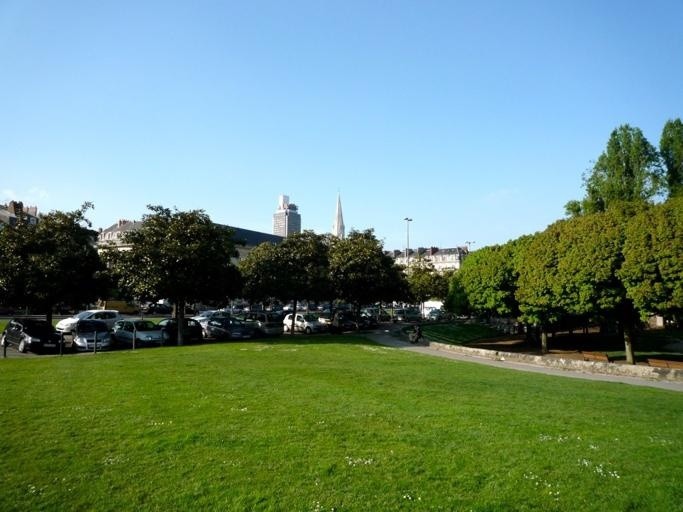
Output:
[464,240,476,254]
[403,216,413,276]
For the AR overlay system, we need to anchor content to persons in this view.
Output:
[407,324,422,344]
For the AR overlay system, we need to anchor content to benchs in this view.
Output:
[647,358,683,369]
[582,351,609,362]
